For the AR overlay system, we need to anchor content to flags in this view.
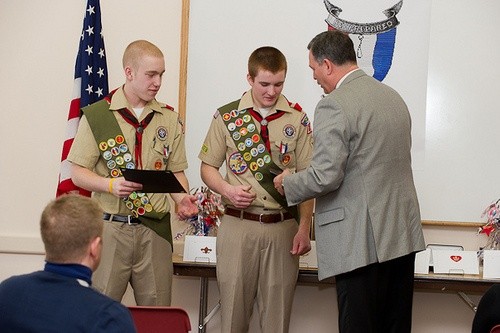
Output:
[56,0,111,199]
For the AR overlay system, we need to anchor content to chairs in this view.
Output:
[127,306,191,333]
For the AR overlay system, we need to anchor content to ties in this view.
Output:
[247,108,285,161]
[103,88,155,170]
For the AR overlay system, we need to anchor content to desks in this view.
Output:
[171,261,500,333]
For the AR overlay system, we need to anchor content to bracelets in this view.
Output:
[281,179,284,190]
[109,178,116,193]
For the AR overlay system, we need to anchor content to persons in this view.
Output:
[0,194,136,333]
[271,30,424,332]
[198,47,315,333]
[66,41,199,306]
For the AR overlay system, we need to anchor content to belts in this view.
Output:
[224,206,296,223]
[103,212,141,225]
